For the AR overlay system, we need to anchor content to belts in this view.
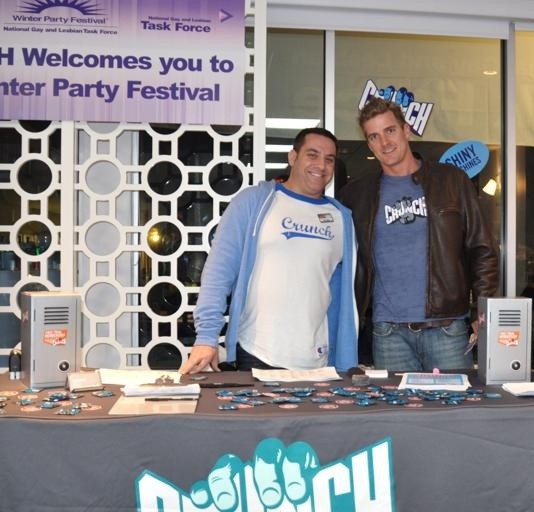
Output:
[384,319,452,332]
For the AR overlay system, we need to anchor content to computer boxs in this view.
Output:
[477,296,533,386]
[20,291,81,390]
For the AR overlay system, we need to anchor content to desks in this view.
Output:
[0,367,534,511]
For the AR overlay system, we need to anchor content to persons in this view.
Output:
[275,97,500,372]
[178,127,360,376]
[190,437,320,512]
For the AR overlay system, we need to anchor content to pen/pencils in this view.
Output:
[199,382,254,388]
[145,397,199,401]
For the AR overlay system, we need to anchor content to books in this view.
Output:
[398,372,472,392]
[119,383,201,397]
